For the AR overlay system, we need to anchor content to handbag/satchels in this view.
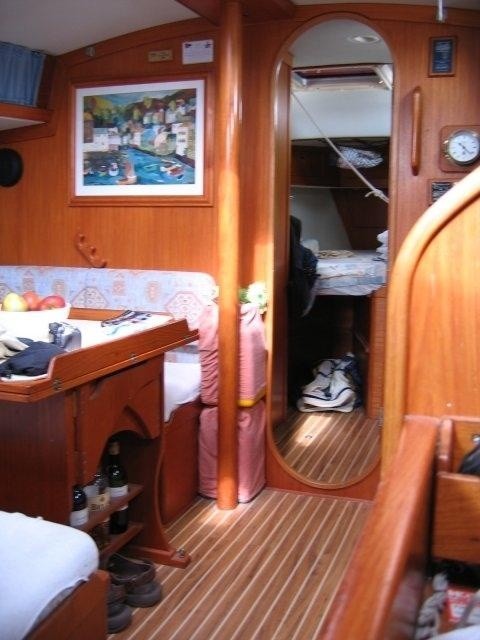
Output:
[0,332,69,380]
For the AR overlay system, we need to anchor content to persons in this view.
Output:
[288,215,318,318]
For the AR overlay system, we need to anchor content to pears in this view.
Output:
[2,293,28,311]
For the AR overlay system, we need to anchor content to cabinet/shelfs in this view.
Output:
[0,303,201,603]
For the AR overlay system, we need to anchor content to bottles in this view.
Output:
[110,441,128,534]
[88,473,109,546]
[71,485,88,534]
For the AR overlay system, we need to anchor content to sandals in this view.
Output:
[94,552,164,632]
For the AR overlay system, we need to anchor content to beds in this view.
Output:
[0,507,111,639]
[309,248,387,299]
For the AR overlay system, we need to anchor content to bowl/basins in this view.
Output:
[0,302,70,351]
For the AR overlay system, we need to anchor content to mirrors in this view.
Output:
[267,12,396,491]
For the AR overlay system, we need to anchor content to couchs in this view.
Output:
[0,264,218,523]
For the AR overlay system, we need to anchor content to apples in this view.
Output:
[39,296,65,310]
[22,291,40,311]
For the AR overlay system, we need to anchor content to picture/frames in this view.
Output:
[66,71,216,209]
[428,178,460,206]
[428,35,458,77]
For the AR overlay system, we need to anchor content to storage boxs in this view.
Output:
[199,303,266,502]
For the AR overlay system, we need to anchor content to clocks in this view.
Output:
[439,124,480,173]
